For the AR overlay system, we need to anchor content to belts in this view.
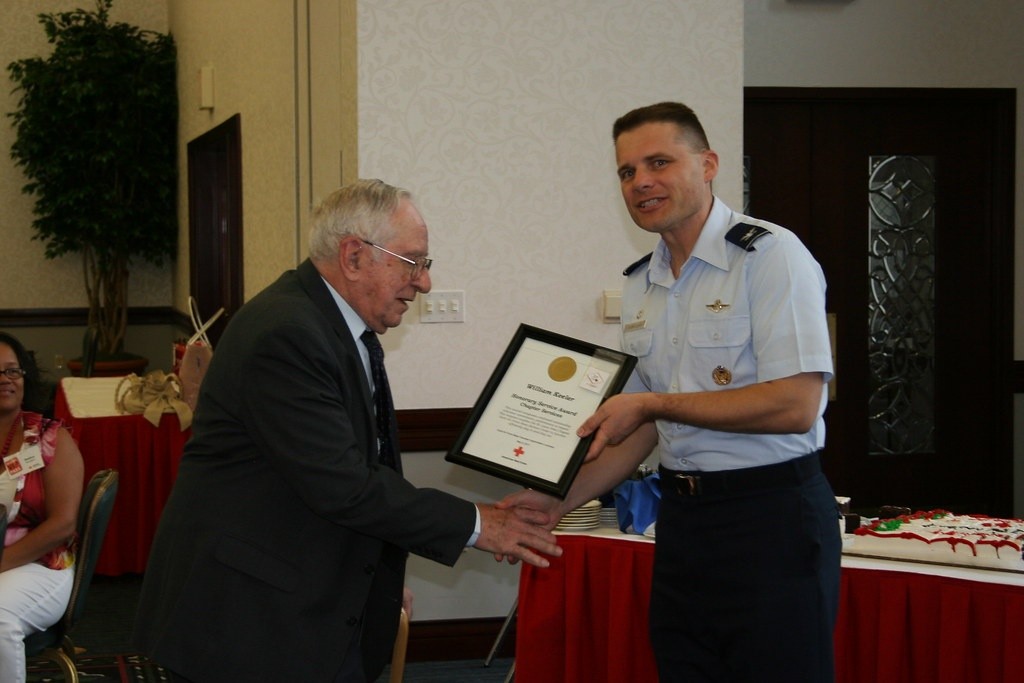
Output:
[658,452,821,498]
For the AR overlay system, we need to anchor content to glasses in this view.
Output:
[0,368,26,379]
[360,238,432,281]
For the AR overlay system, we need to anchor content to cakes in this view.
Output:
[854,509,1024,570]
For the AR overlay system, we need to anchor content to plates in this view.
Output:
[554,488,618,534]
[643,532,655,539]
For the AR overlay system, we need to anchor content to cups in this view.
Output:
[171,341,202,375]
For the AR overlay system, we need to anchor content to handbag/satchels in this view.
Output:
[178,295,224,413]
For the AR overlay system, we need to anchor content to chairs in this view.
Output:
[22,467,120,683]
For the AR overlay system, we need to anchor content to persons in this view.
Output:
[124,176,566,683]
[492,99,844,683]
[0,329,87,683]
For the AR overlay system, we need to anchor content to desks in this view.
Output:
[54,377,192,575]
[514,517,1024,683]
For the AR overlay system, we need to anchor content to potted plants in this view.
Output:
[4,0,180,377]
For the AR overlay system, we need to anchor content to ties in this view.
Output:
[360,330,393,473]
